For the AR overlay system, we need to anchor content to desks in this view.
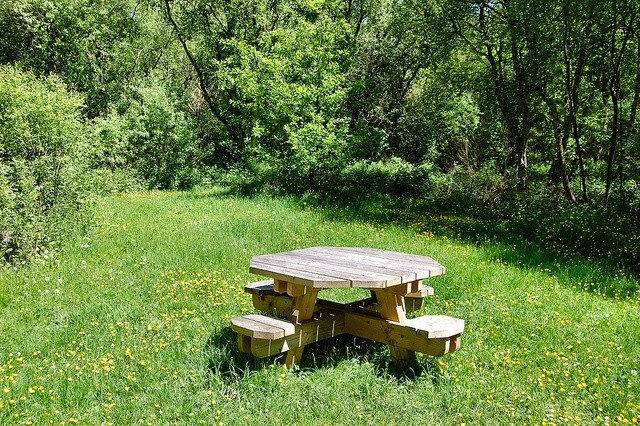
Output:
[249,245,446,376]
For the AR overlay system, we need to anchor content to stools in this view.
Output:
[404,284,435,313]
[405,314,465,357]
[229,314,296,358]
[243,279,287,315]
